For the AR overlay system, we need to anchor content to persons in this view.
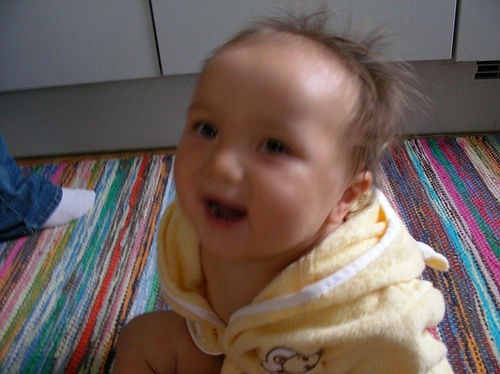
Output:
[0,128,96,244]
[109,9,455,374]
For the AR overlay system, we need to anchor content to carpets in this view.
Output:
[0,133,500,374]
